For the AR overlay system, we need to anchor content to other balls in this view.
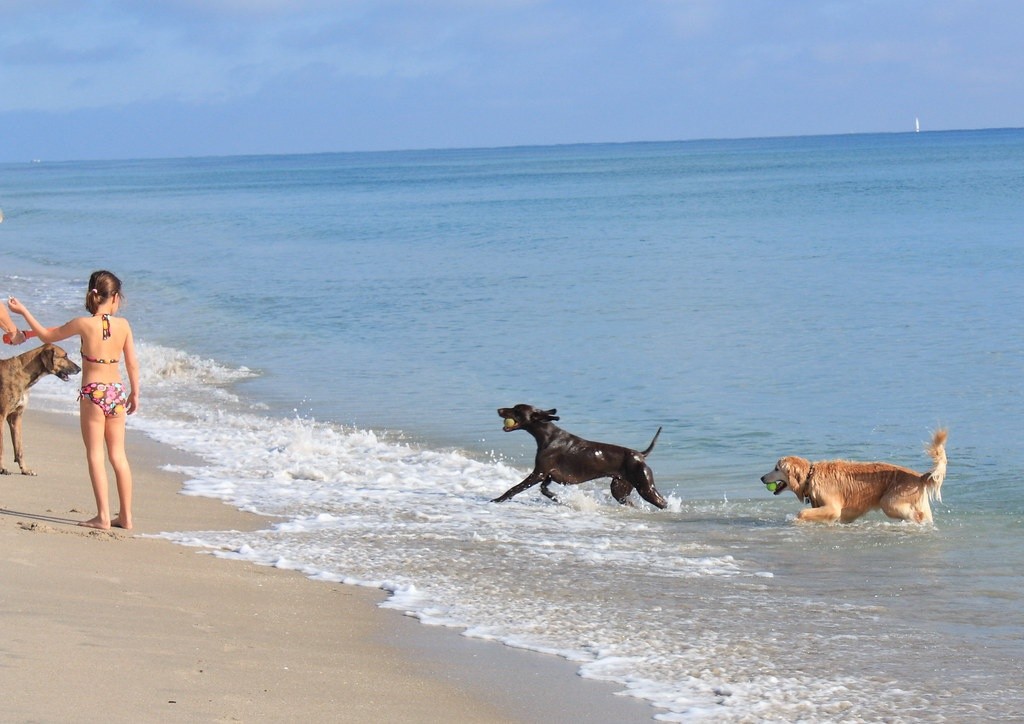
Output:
[766,481,777,491]
[504,418,515,428]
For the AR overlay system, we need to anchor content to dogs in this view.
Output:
[0,343,82,476]
[759,427,951,529]
[489,403,673,514]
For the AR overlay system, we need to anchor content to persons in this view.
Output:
[7,270,139,529]
[0,300,26,346]
